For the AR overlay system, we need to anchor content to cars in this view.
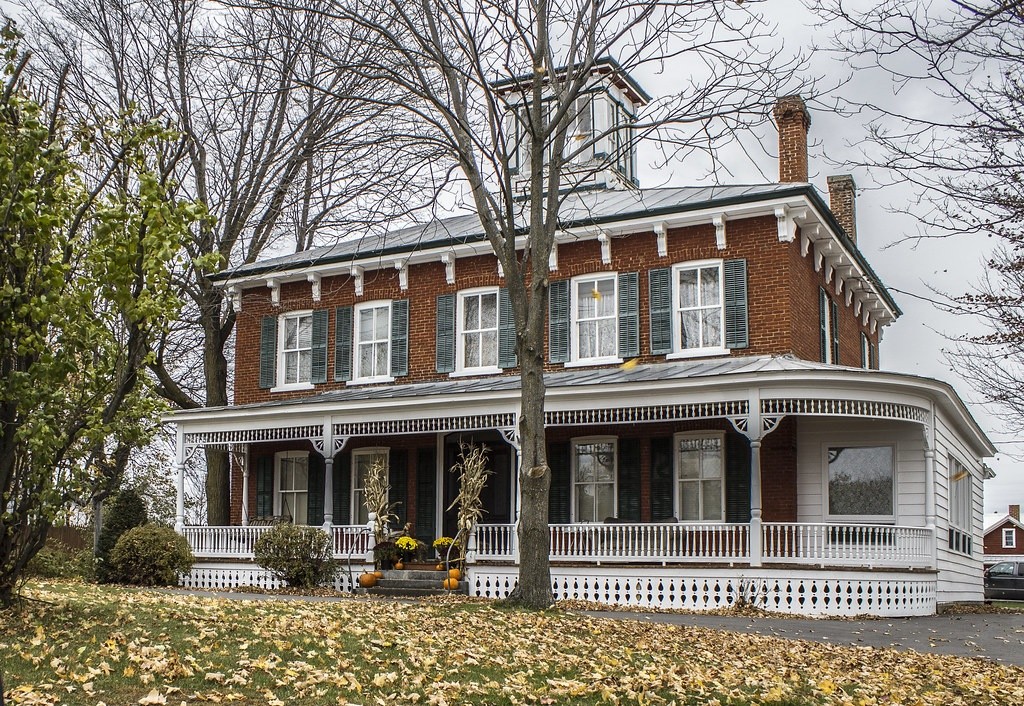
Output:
[983,561,1024,603]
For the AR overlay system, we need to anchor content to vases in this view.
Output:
[381,560,393,570]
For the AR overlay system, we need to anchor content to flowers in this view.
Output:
[395,536,418,555]
[431,537,461,562]
[373,542,395,559]
